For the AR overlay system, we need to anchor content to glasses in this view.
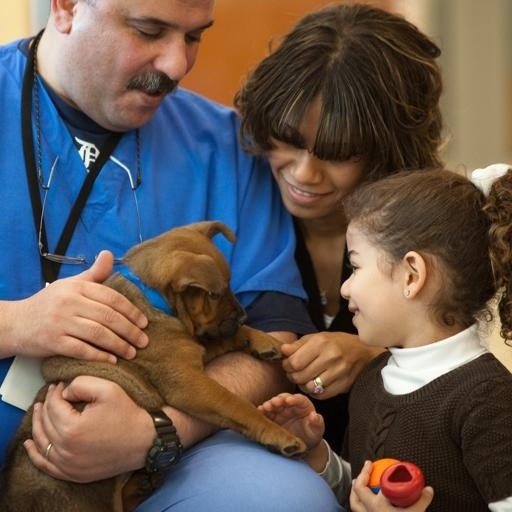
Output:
[37,154,144,266]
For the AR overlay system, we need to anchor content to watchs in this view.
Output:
[147,405,184,478]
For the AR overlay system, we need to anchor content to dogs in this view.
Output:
[0,220,308,512]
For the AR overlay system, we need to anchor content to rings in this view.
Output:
[46,442,53,460]
[313,376,324,394]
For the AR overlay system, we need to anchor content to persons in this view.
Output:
[0,0,345,511]
[253,169,512,512]
[232,1,448,459]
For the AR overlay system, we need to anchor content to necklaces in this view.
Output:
[320,285,328,306]
